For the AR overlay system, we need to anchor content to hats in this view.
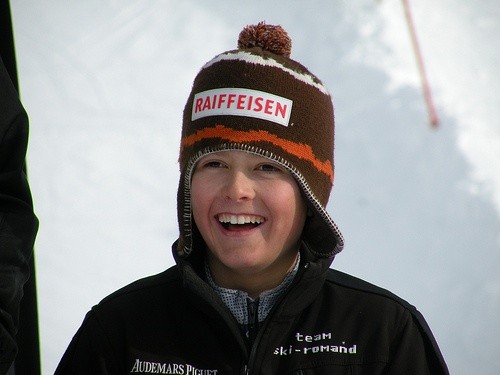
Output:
[172,20,344,263]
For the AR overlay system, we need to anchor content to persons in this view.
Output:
[53,22,450,375]
